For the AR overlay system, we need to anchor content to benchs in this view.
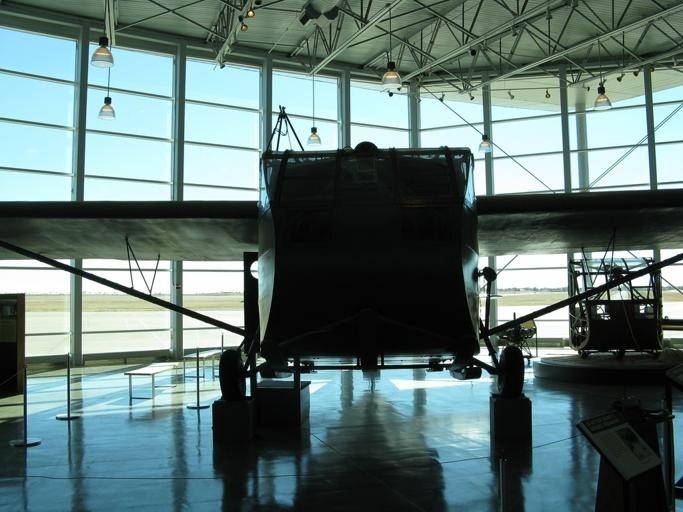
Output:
[123,346,223,408]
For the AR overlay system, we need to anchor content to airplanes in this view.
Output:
[0,104,682,400]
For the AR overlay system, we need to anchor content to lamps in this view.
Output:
[89,1,117,69]
[305,65,324,150]
[97,45,118,122]
[477,91,494,150]
[591,39,613,114]
[378,7,405,90]
